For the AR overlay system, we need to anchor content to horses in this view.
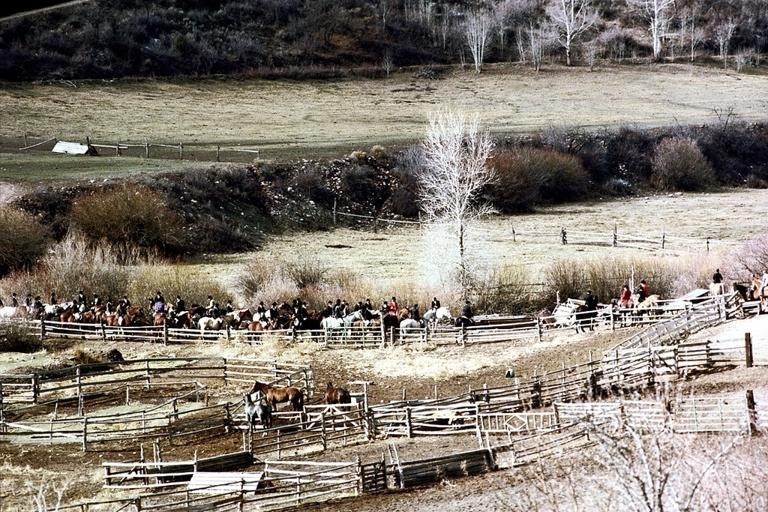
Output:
[710,275,768,314]
[242,382,305,431]
[565,293,663,334]
[324,380,351,429]
[1,301,474,348]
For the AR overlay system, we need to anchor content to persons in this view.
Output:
[620,285,632,302]
[559,229,568,246]
[381,296,420,323]
[733,282,748,302]
[256,297,374,327]
[711,269,723,284]
[24,290,132,333]
[585,290,598,311]
[149,292,236,322]
[637,280,649,303]
[431,296,440,310]
[463,301,473,318]
[12,293,18,308]
